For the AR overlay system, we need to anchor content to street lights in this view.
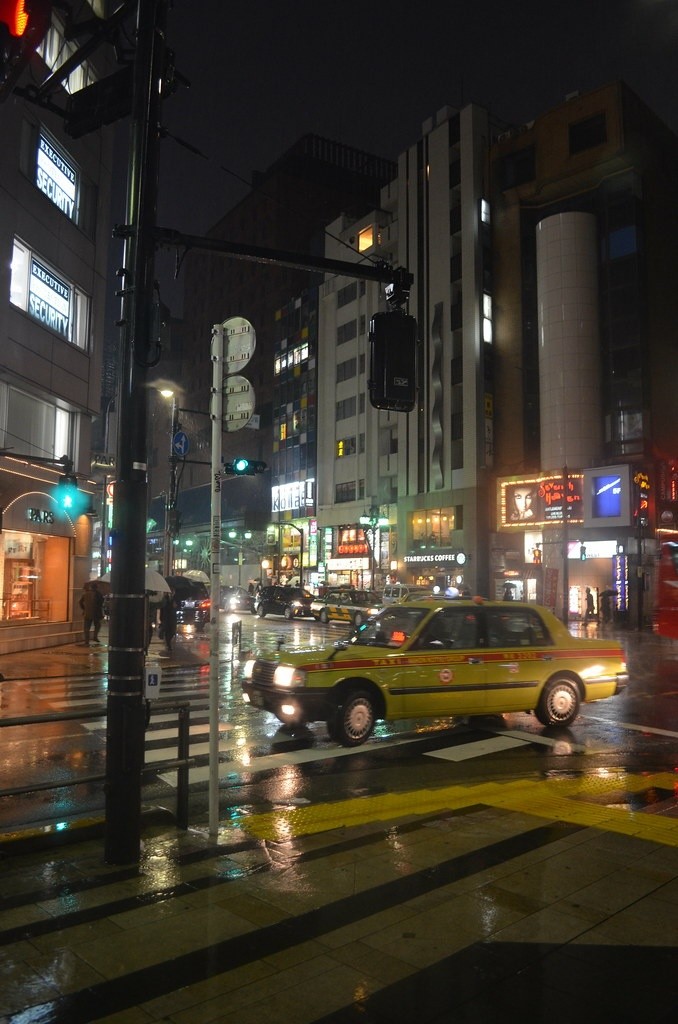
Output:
[358,505,390,591]
[172,537,193,576]
[227,529,253,587]
[267,521,304,589]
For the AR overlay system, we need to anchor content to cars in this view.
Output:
[217,583,256,614]
[238,595,629,748]
[253,585,316,621]
[310,590,386,627]
[382,584,431,605]
[165,577,211,631]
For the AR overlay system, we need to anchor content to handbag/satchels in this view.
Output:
[157,622,165,640]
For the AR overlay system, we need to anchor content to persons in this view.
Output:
[248,583,254,596]
[503,588,513,601]
[600,596,612,624]
[255,582,263,594]
[147,591,161,646]
[78,584,105,646]
[509,485,537,522]
[582,587,601,627]
[158,589,179,650]
[295,580,299,586]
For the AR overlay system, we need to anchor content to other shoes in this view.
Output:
[85,641,89,645]
[93,638,101,642]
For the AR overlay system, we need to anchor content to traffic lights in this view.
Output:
[57,474,79,511]
[232,459,270,476]
[580,546,586,562]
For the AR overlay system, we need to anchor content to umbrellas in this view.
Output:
[502,583,517,588]
[247,579,256,583]
[598,589,618,597]
[254,577,260,581]
[100,568,172,593]
[184,568,210,583]
[165,576,210,599]
